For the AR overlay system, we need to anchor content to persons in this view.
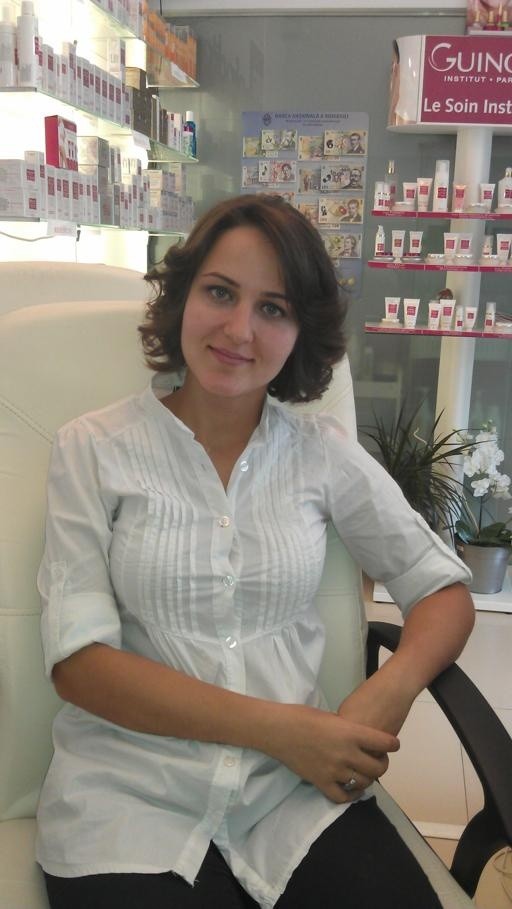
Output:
[281,164,294,182]
[280,130,295,150]
[340,168,363,191]
[386,39,414,125]
[282,194,291,205]
[35,193,474,909]
[338,235,358,258]
[344,132,365,154]
[340,200,362,222]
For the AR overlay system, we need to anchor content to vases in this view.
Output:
[452,527,512,597]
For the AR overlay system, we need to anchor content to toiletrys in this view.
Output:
[472,2,511,31]
[372,157,512,333]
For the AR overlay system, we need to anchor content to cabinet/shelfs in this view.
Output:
[361,204,510,341]
[0,0,203,238]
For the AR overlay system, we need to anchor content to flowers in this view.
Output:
[446,414,512,553]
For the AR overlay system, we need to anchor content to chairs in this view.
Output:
[2,288,511,909]
[0,258,168,327]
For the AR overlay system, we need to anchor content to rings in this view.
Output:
[343,767,356,790]
